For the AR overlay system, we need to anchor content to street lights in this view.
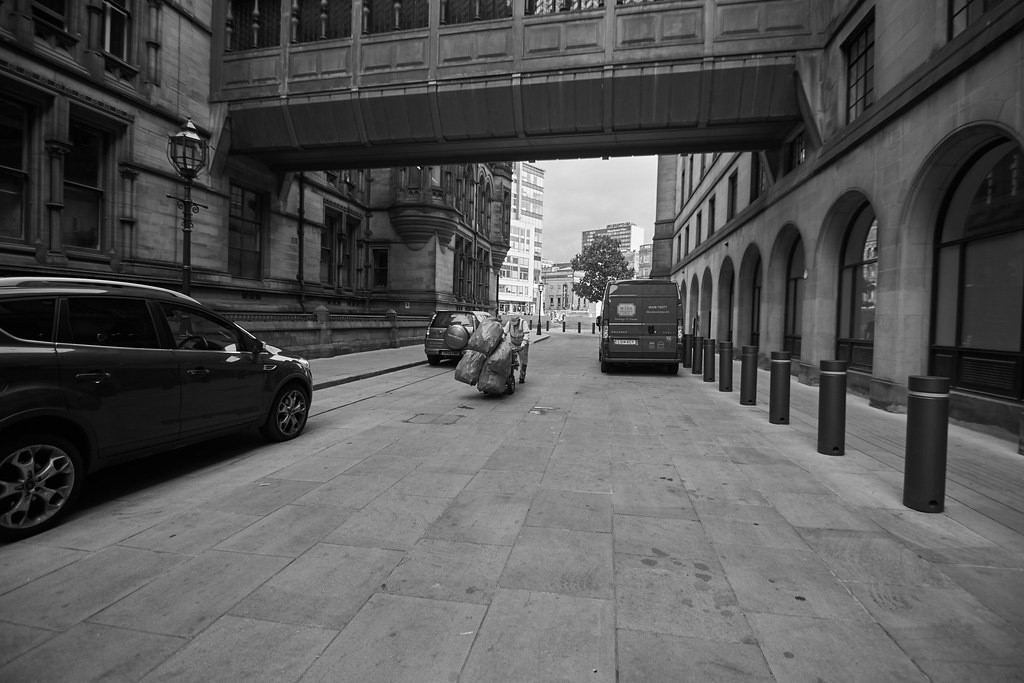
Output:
[495,264,500,318]
[537,283,544,335]
[166,115,210,337]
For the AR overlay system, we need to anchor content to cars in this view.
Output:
[0,275,313,533]
[425,310,494,365]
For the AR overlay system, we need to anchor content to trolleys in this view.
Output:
[478,347,518,394]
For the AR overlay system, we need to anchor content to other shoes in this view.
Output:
[519,378,524,383]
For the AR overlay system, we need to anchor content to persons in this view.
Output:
[501,315,530,383]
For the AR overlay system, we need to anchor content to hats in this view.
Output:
[511,315,519,325]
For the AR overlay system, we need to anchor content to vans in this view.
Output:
[596,277,682,375]
